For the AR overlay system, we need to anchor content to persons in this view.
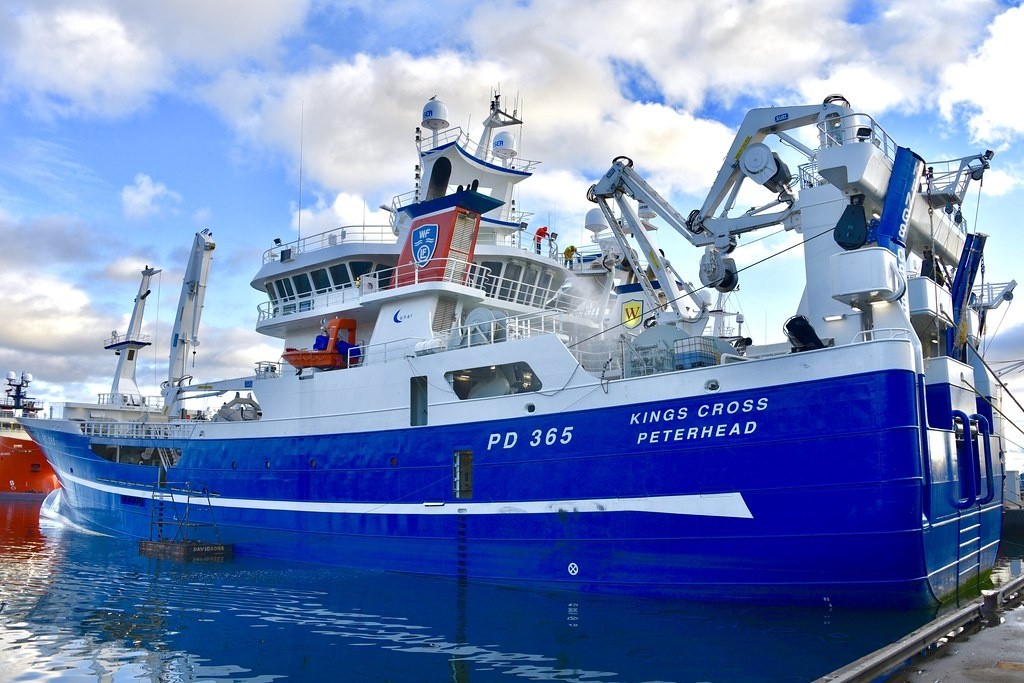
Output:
[533,227,549,255]
[564,246,581,269]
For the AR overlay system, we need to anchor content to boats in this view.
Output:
[281,316,358,371]
[0,369,62,501]
[10,87,1023,614]
